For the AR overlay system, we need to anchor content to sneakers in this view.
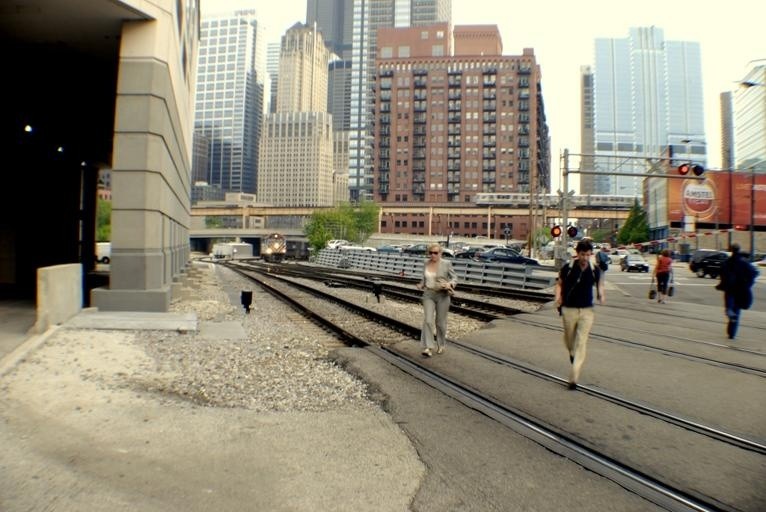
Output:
[422,348,433,357]
[436,346,444,354]
[569,383,577,390]
[728,320,737,339]
[570,355,574,364]
[658,298,667,304]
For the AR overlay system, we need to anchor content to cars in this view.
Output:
[689,248,733,279]
[543,237,649,273]
[327,239,539,266]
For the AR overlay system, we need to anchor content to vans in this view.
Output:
[95,242,111,264]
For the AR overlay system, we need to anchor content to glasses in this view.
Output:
[428,251,440,255]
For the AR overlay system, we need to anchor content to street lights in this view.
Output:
[681,138,707,145]
[740,80,766,88]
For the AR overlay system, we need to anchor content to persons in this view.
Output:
[555,241,604,390]
[715,242,758,339]
[417,244,457,357]
[653,250,672,304]
[595,247,609,303]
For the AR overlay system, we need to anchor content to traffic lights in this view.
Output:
[679,164,704,176]
[551,225,577,237]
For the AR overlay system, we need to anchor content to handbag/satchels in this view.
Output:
[649,280,657,300]
[598,251,608,271]
[668,283,675,297]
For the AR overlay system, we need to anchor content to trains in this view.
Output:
[474,193,644,207]
[260,232,310,263]
